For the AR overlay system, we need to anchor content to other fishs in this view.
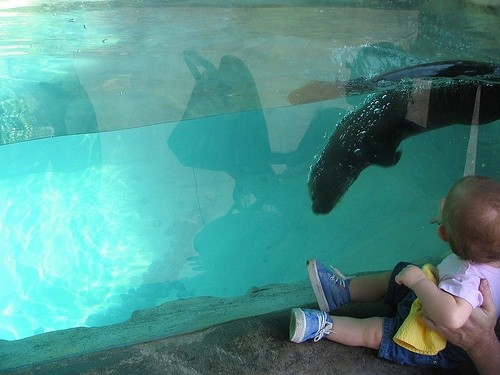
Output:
[307,58,500,213]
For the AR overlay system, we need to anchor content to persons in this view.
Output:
[416,279,500,375]
[290,174,500,369]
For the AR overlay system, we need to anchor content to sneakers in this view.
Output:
[289,308,336,343]
[307,260,351,313]
